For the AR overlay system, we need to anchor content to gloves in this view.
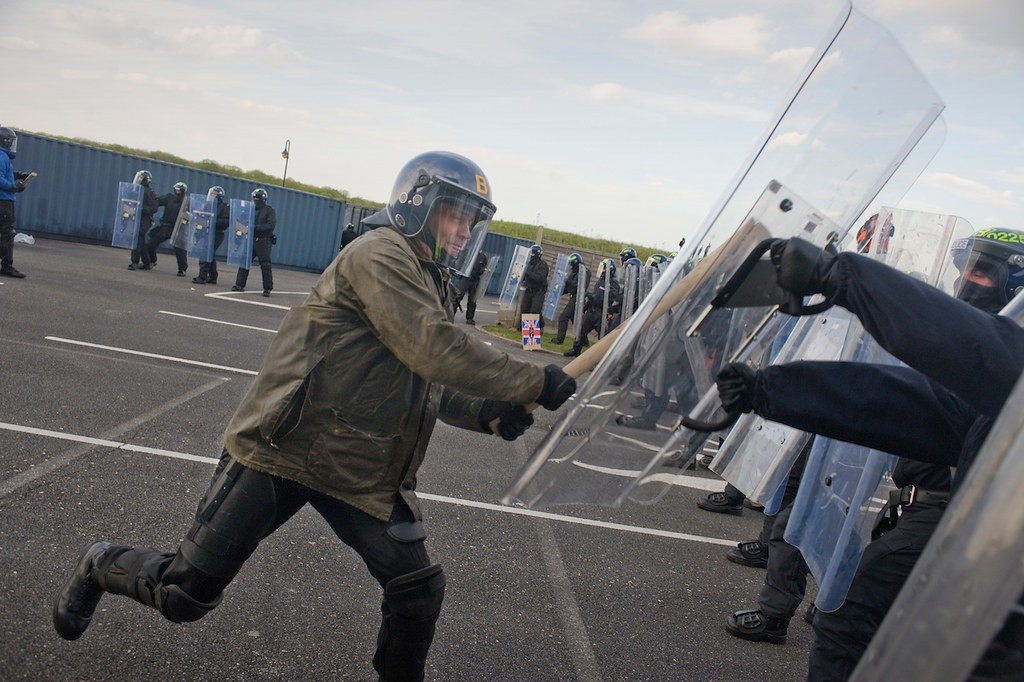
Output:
[716,361,754,414]
[497,404,534,441]
[777,236,835,294]
[535,363,577,411]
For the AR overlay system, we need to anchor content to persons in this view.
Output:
[144,182,189,276]
[338,222,358,252]
[123,170,158,271]
[192,187,230,284]
[53,149,578,681]
[231,188,277,297]
[449,242,488,324]
[550,227,1024,682]
[0,126,29,279]
[516,245,549,337]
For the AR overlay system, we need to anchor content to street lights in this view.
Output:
[281,140,291,187]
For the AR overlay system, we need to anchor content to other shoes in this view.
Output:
[466,319,475,324]
[8,179,26,194]
[192,276,217,284]
[608,376,788,644]
[231,283,245,291]
[0,264,26,278]
[263,289,271,297]
[13,170,29,181]
[128,261,158,270]
[540,326,589,357]
[177,269,186,276]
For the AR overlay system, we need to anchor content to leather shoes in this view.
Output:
[53,541,119,641]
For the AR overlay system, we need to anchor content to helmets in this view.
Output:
[0,127,18,160]
[529,244,688,278]
[360,151,497,268]
[251,188,268,208]
[137,170,152,186]
[209,186,225,204]
[173,182,188,196]
[950,227,1024,312]
[346,223,355,231]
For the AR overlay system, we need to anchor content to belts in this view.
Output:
[871,485,950,541]
